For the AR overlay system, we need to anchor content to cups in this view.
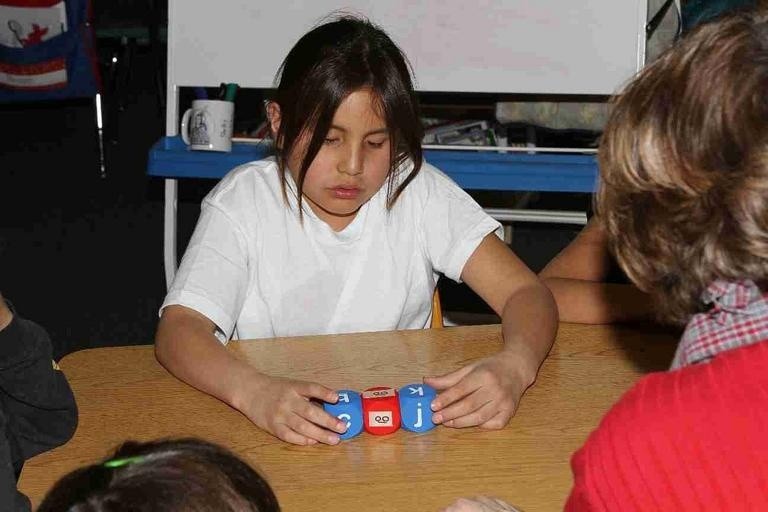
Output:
[182,99,234,154]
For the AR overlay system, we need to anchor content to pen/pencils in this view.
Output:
[196,82,239,101]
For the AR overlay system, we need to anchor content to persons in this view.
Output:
[36,433,282,511]
[443,2,768,512]
[153,15,558,445]
[0,292,80,511]
[534,212,664,325]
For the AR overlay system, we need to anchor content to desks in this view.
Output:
[15,321,678,512]
